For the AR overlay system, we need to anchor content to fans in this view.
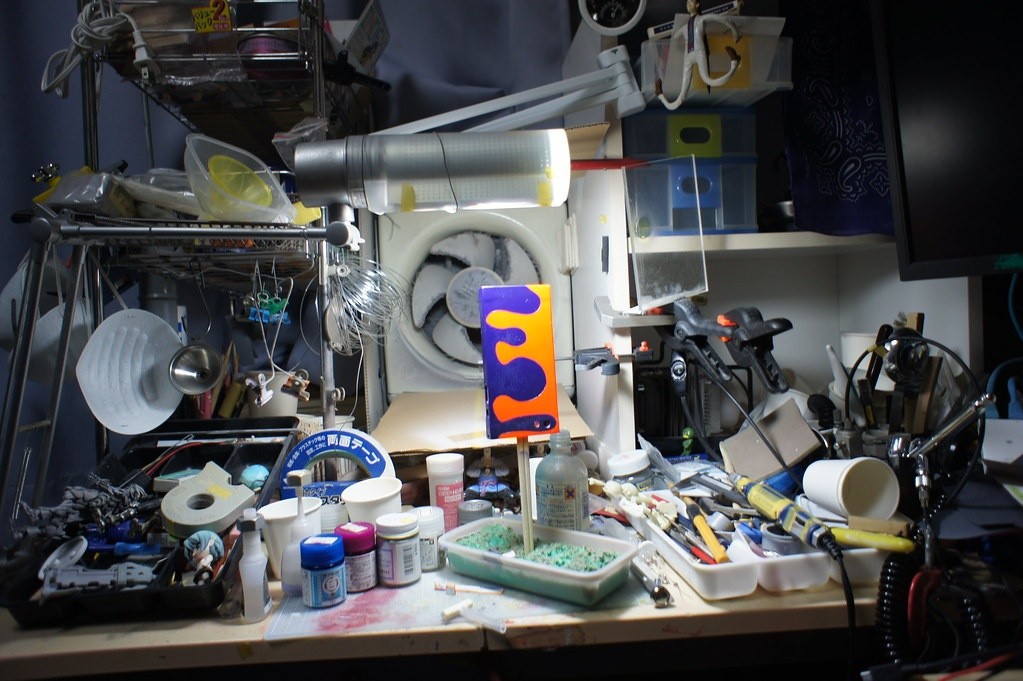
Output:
[378,202,575,400]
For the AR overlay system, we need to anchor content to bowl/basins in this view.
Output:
[183,133,292,221]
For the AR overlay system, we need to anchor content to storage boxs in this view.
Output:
[628,158,762,233]
[639,35,794,113]
[436,518,639,605]
[620,109,755,158]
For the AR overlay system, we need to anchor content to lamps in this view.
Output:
[0,127,573,557]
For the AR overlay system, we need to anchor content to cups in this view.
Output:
[803,457,899,519]
[257,496,322,578]
[340,476,403,526]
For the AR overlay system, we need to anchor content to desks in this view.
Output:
[0,508,887,681]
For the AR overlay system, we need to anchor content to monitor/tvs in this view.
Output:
[868,0,1023,282]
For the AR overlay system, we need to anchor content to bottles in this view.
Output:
[411,506,447,571]
[607,449,654,493]
[300,533,347,608]
[534,429,589,531]
[457,500,492,526]
[238,508,272,621]
[320,504,348,534]
[426,453,465,533]
[281,487,314,598]
[334,521,378,592]
[375,513,422,587]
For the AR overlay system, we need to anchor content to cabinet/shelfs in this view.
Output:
[75,0,373,498]
